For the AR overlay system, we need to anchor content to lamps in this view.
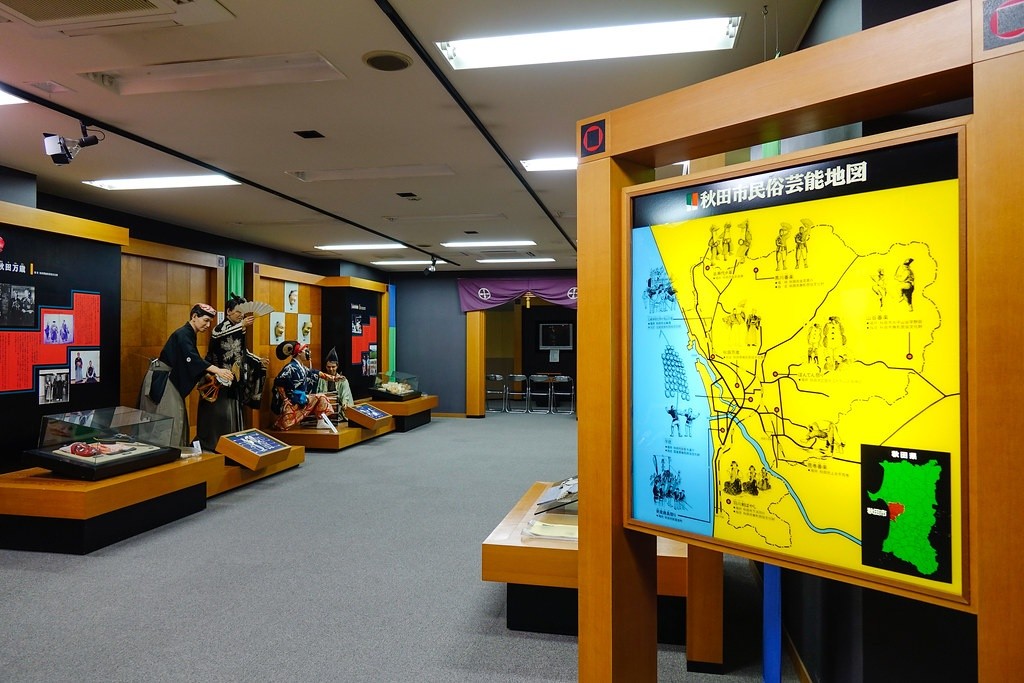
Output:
[43,118,105,166]
[423,255,437,279]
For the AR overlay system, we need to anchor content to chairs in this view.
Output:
[485,374,574,415]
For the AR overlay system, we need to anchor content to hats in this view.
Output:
[327,347,338,364]
[293,344,307,355]
[197,303,217,315]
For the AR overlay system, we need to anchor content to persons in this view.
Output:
[45,375,64,402]
[197,292,269,452]
[0,288,36,326]
[75,352,98,383]
[135,303,234,447]
[270,343,345,432]
[317,347,354,421]
[44,319,70,343]
